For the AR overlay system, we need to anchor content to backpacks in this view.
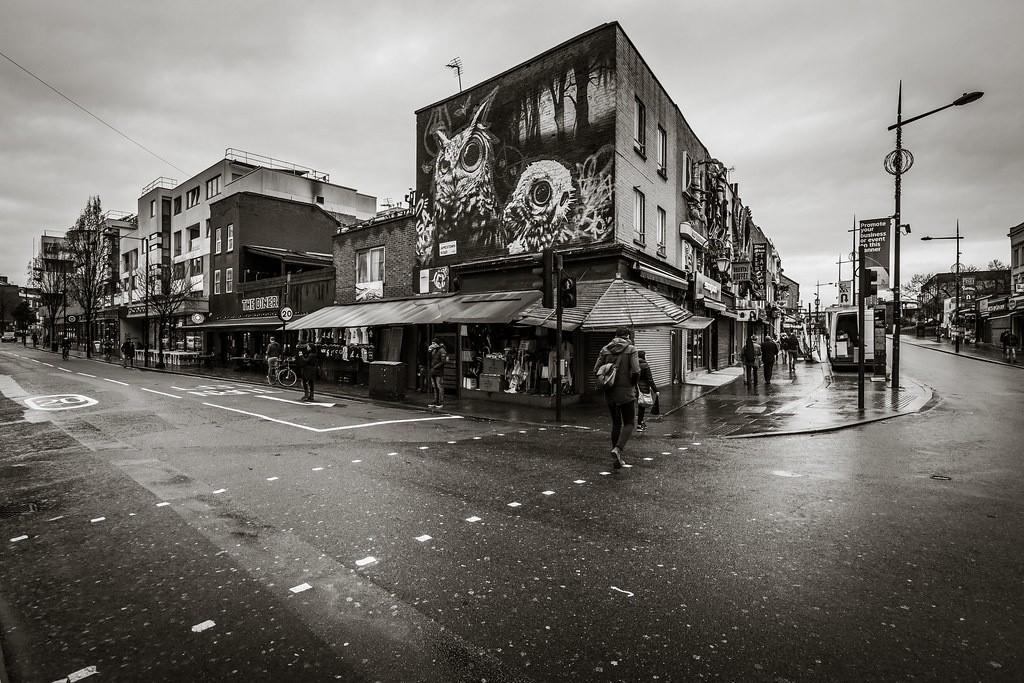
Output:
[746,338,755,362]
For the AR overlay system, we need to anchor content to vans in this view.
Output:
[826,306,875,367]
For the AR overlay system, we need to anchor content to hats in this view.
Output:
[432,338,440,345]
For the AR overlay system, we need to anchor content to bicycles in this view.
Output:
[267,358,298,387]
[62,347,72,361]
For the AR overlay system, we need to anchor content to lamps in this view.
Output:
[700,239,731,274]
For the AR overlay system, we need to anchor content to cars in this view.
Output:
[2,332,18,343]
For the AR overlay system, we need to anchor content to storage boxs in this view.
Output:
[479,357,509,393]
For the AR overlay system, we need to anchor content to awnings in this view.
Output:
[181,314,304,331]
[276,291,543,333]
[525,271,691,341]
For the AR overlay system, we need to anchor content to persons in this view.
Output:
[60,335,71,360]
[741,331,801,387]
[593,326,640,467]
[1000,330,1019,361]
[636,351,660,432]
[121,338,136,368]
[299,343,317,401]
[102,339,114,359]
[265,336,281,382]
[296,340,309,386]
[426,338,447,409]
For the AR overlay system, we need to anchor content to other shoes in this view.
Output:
[436,404,443,407]
[308,396,314,400]
[766,380,770,384]
[642,422,648,428]
[301,395,309,400]
[428,401,438,406]
[747,385,752,389]
[754,382,758,387]
[611,446,622,468]
[637,425,646,432]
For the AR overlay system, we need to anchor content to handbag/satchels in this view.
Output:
[650,396,660,415]
[595,363,618,390]
[638,392,653,408]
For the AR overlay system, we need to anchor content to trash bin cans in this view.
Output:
[916,325,925,338]
[52,341,59,351]
[368,361,407,400]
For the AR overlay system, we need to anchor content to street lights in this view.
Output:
[814,280,833,323]
[5,285,28,346]
[882,78,986,388]
[101,231,149,367]
[921,217,965,353]
[32,267,66,335]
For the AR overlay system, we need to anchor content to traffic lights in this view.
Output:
[560,277,577,308]
[531,248,555,309]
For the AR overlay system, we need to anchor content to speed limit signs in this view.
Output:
[280,306,294,322]
